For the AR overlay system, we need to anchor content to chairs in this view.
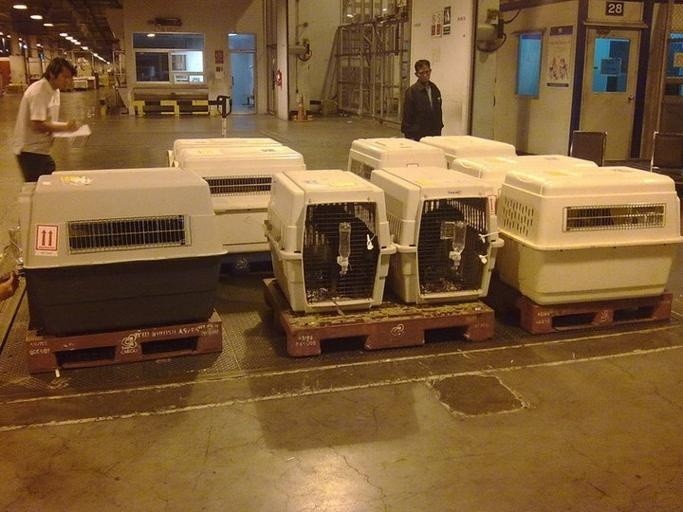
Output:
[570,130,682,185]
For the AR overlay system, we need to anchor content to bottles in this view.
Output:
[7,214,24,276]
[339,223,351,273]
[452,221,466,266]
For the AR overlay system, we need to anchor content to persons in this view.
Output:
[400,58,444,143]
[9,58,83,186]
